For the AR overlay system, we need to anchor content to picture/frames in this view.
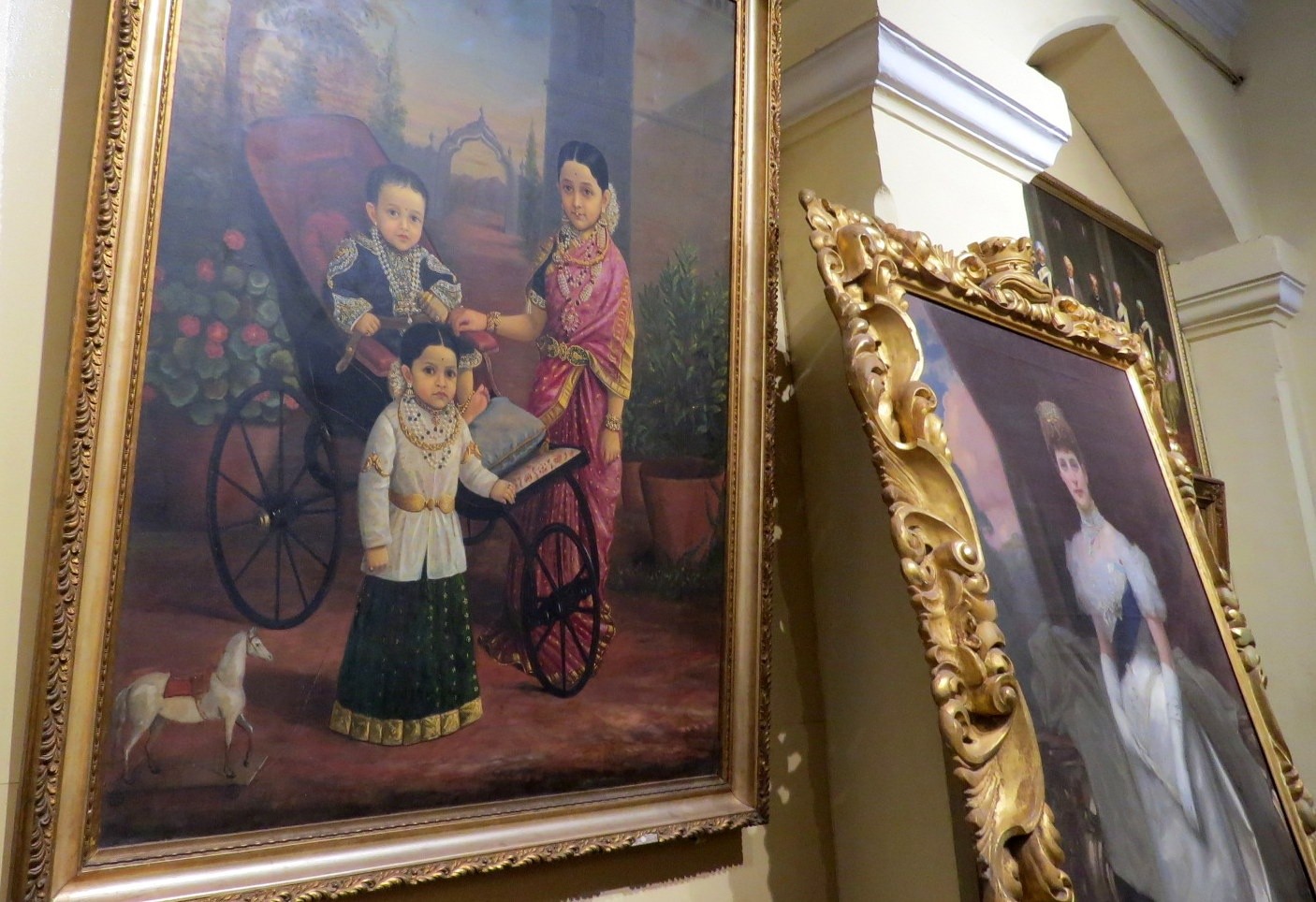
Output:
[5,2,795,901]
[1023,171,1210,477]
[800,181,1316,900]
